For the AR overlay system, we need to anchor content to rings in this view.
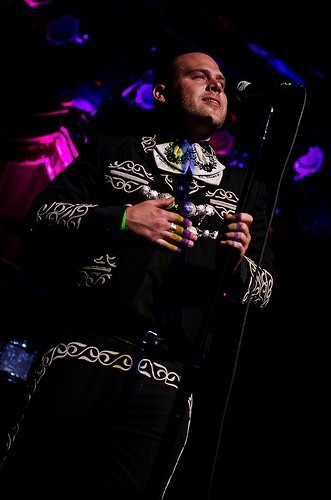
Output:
[170,222,176,232]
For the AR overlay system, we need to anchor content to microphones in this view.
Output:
[236,80,304,102]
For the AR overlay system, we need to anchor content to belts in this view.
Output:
[123,327,170,354]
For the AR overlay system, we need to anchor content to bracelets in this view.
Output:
[122,209,127,230]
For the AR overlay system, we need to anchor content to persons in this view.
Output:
[0,51,275,500]
